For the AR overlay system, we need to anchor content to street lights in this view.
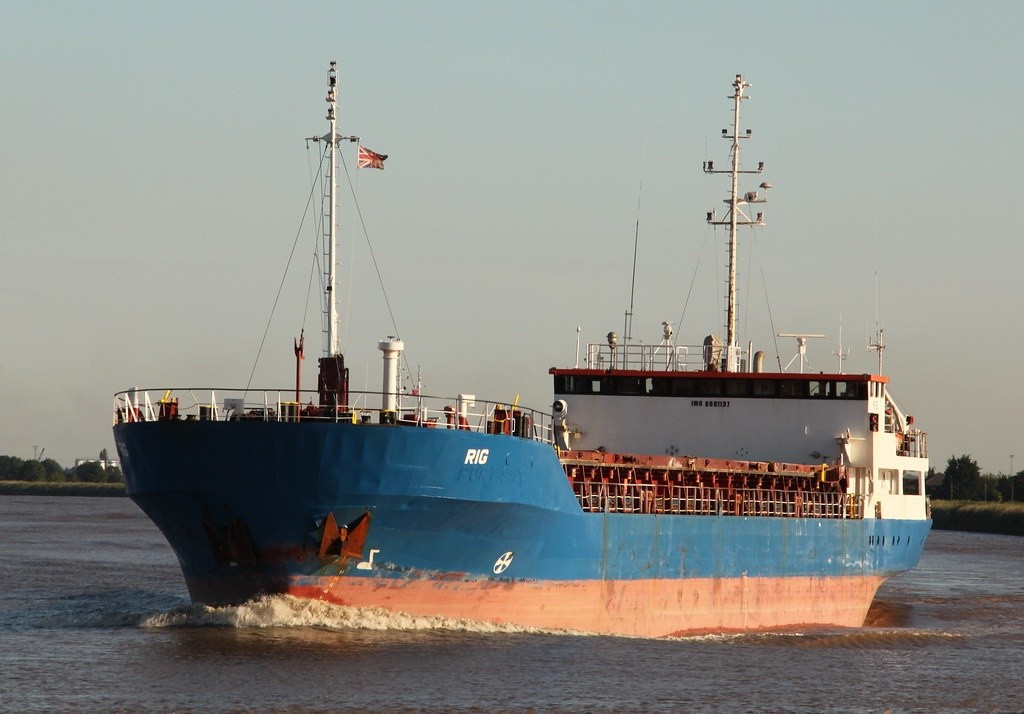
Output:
[1009,454,1015,503]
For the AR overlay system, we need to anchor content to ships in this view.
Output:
[111,56,934,651]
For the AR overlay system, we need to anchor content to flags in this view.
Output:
[358,145,388,171]
